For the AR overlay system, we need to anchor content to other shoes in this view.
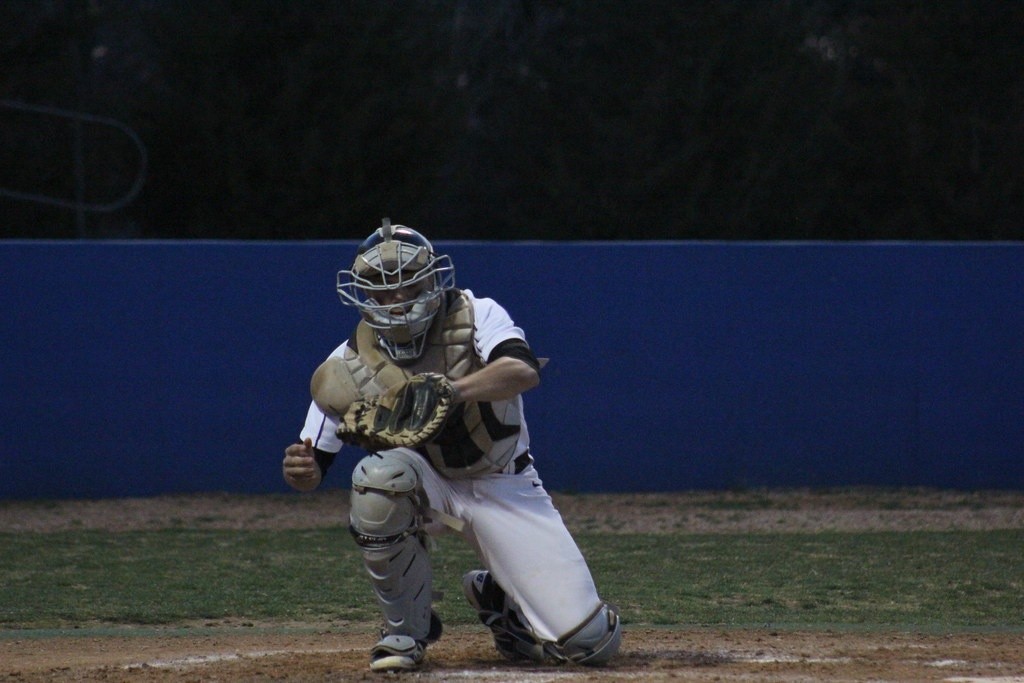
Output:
[370,629,425,671]
[463,570,537,664]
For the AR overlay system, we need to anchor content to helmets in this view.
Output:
[336,218,455,368]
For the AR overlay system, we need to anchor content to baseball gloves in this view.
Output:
[334,372,457,451]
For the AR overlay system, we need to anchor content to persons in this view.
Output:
[282,223,621,671]
